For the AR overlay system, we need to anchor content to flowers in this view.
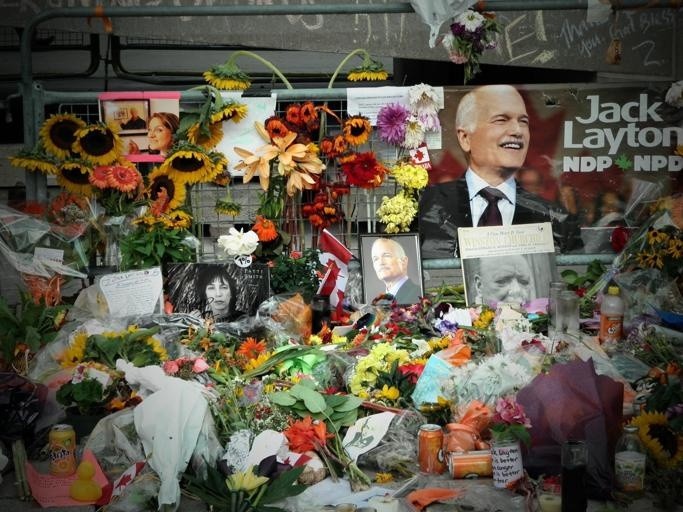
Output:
[440,0,501,83]
[574,192,682,504]
[0,49,570,506]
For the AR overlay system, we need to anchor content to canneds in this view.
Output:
[418,424,446,474]
[48,424,76,476]
[449,450,493,481]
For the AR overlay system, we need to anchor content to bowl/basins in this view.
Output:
[579,224,641,253]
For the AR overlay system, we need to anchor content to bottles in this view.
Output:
[598,284,626,346]
[548,281,581,339]
[613,424,650,495]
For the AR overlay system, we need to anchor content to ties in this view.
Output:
[476,187,504,227]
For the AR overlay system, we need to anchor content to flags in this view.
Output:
[316,228,354,312]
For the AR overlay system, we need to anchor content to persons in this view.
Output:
[473,253,533,308]
[515,163,661,224]
[183,265,249,324]
[416,85,583,262]
[119,107,146,129]
[370,238,421,304]
[128,112,179,173]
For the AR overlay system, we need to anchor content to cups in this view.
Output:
[533,482,563,512]
[558,438,590,512]
